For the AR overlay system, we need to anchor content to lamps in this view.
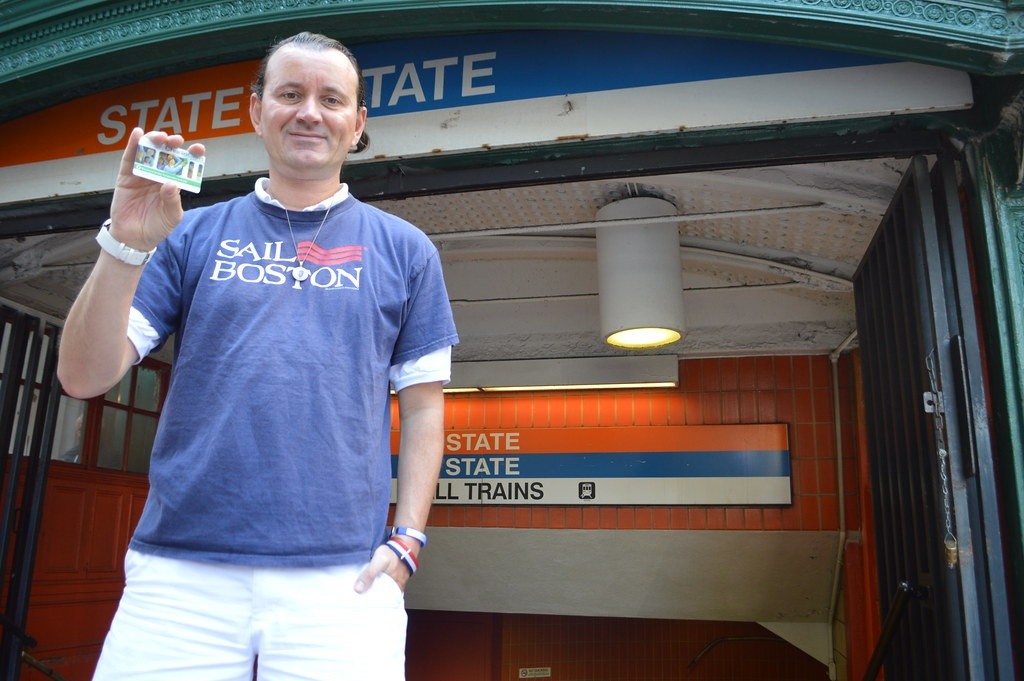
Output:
[595,196,688,350]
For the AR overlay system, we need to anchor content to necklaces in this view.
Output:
[285,206,332,282]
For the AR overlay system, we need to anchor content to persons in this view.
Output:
[55,31,462,681]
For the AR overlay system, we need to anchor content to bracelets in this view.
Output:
[385,536,419,576]
[95,217,158,266]
[388,525,427,548]
